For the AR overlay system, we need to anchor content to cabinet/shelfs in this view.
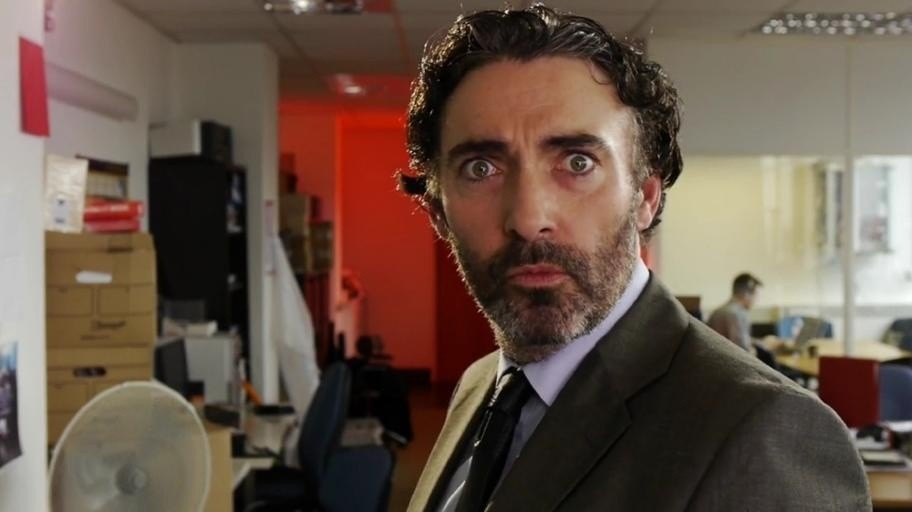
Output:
[149,156,251,403]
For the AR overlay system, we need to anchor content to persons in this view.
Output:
[706,271,780,375]
[391,0,875,512]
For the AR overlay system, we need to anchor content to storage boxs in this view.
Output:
[279,192,334,272]
[45,231,231,512]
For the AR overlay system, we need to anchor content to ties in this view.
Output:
[452,366,533,512]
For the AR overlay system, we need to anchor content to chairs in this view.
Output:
[231,361,396,512]
[751,323,810,389]
[884,318,912,368]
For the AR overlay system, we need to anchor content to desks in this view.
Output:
[864,470,912,512]
[757,337,912,377]
